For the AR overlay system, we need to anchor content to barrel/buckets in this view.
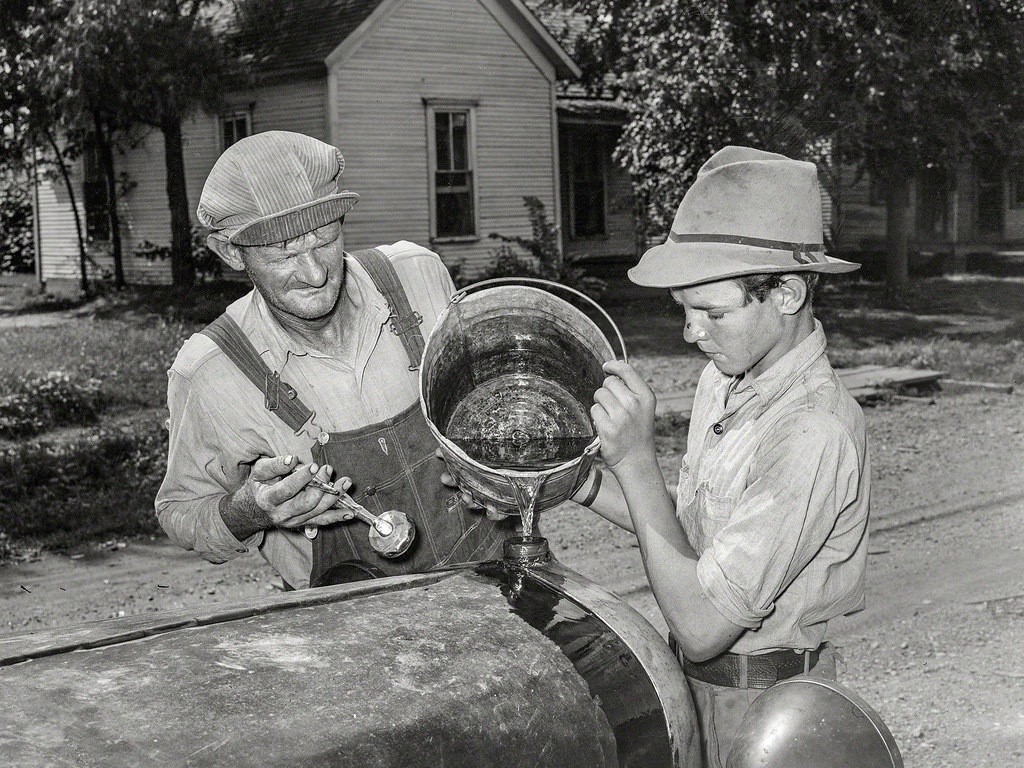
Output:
[417,275,629,517]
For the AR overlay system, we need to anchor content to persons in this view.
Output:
[154,130,558,593]
[434,146,874,768]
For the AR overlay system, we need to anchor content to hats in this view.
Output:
[197,129,361,245]
[627,145,862,288]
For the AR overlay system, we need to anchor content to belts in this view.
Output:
[668,632,820,689]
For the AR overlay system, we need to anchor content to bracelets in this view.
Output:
[580,467,602,507]
